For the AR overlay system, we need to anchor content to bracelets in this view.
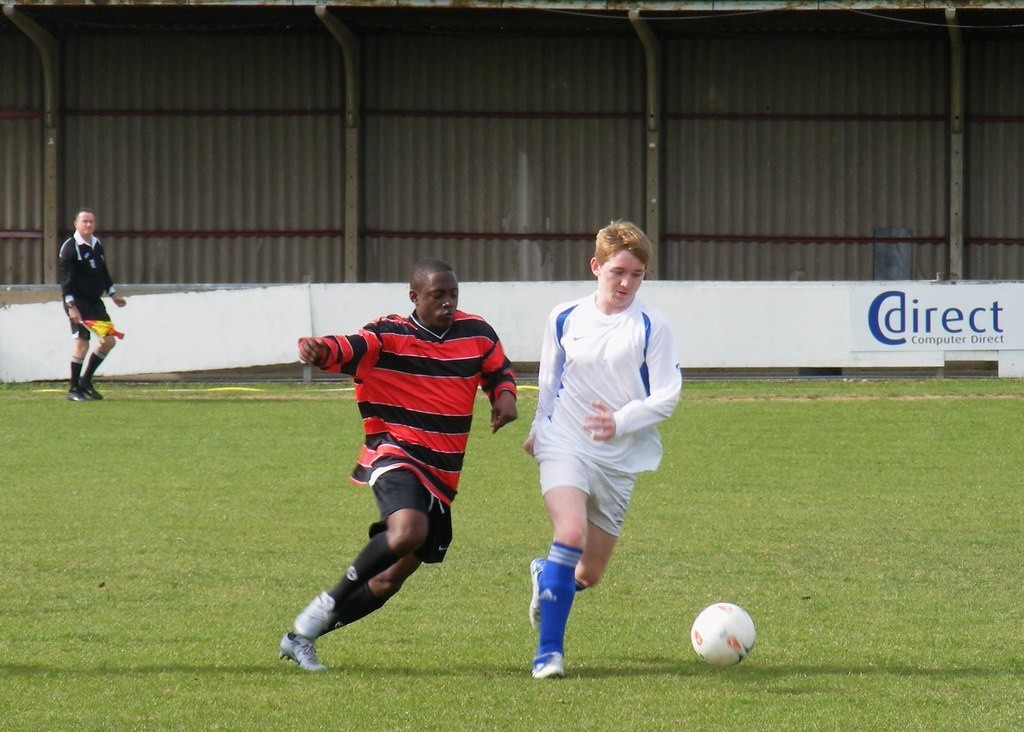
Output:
[67,302,76,309]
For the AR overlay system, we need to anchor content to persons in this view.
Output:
[58,207,126,401]
[522,220,683,680]
[279,257,519,672]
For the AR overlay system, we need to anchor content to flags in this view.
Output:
[83,318,113,338]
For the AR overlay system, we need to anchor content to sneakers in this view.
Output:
[279,632,328,671]
[529,558,548,630]
[531,651,565,680]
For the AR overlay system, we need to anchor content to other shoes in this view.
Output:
[67,391,87,401]
[78,378,103,400]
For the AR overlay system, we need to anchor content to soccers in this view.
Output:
[690,602,756,668]
